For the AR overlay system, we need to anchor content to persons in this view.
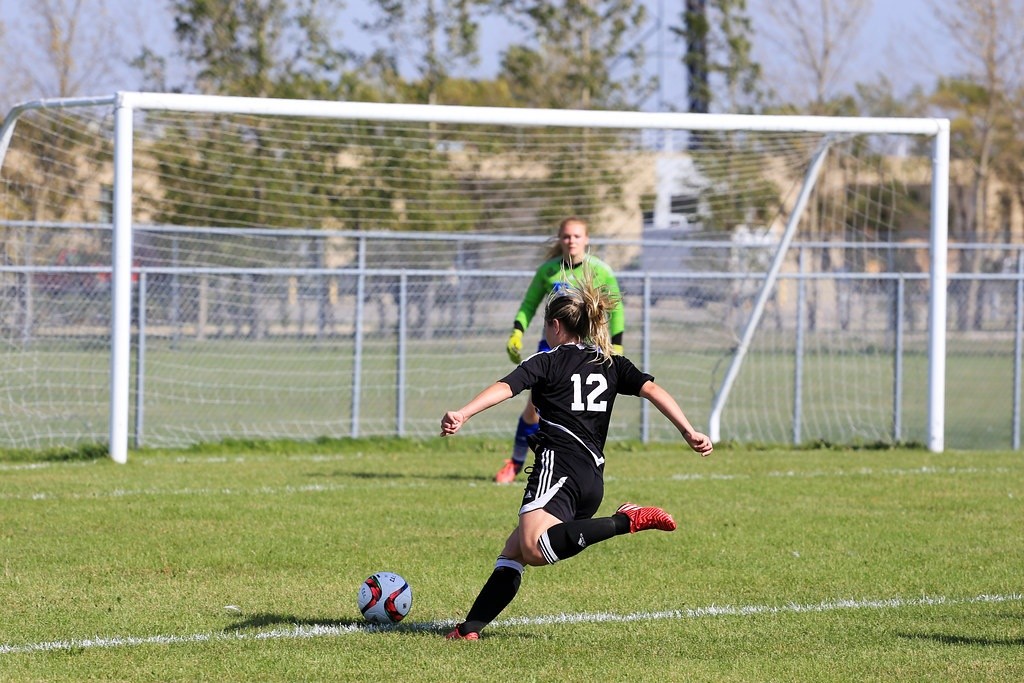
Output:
[494,216,624,483]
[439,290,715,642]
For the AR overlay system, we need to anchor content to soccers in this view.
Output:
[357,571,413,625]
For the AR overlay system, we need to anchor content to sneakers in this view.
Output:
[494,458,523,483]
[617,501,676,534]
[447,623,478,641]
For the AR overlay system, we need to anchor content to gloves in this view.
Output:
[611,344,625,357]
[507,328,524,364]
